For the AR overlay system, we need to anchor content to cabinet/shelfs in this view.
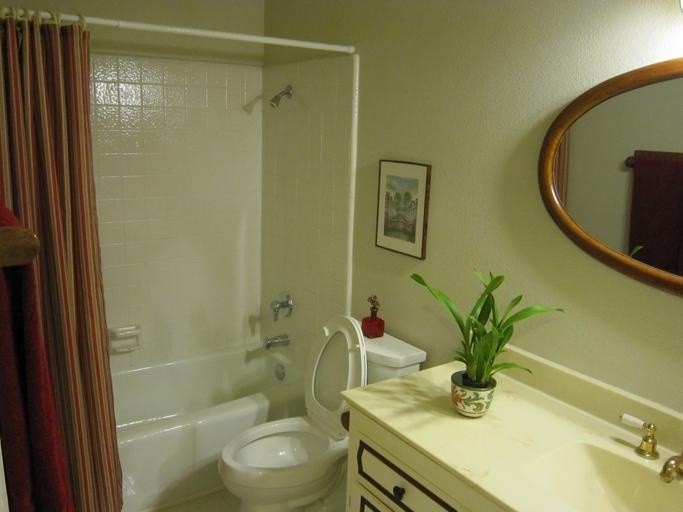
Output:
[344,404,504,511]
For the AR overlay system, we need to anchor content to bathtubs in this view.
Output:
[112,340,304,512]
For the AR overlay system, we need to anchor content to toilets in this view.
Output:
[218,314,426,512]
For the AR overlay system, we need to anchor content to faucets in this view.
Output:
[264,335,289,349]
[659,456,678,483]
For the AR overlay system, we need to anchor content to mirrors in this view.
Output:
[537,57,683,293]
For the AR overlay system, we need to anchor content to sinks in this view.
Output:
[483,442,681,512]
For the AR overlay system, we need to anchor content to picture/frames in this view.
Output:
[375,159,431,260]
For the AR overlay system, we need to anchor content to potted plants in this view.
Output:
[408,267,565,419]
